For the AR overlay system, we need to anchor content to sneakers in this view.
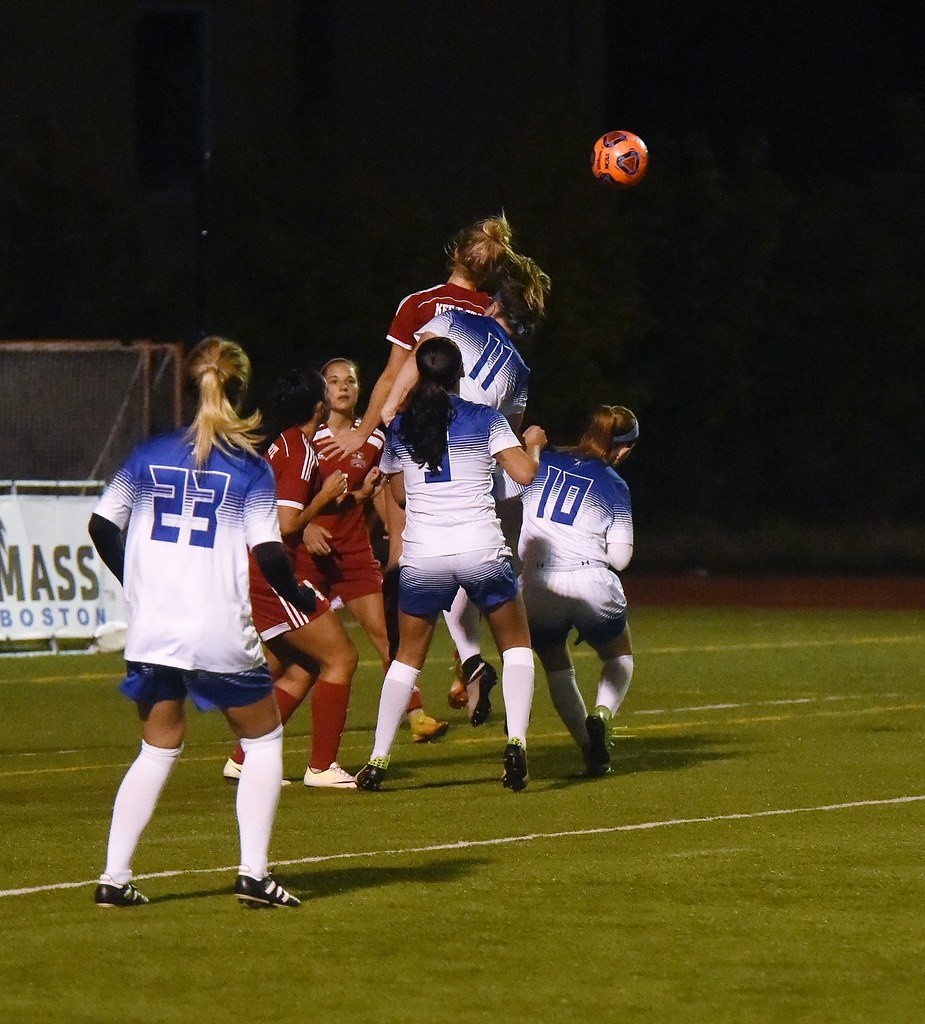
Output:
[410,716,449,743]
[463,660,497,727]
[355,758,391,791]
[223,756,291,787]
[584,706,612,777]
[448,657,469,711]
[235,866,300,908]
[302,760,357,789]
[503,740,531,790]
[94,874,150,909]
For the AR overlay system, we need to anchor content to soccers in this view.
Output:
[590,130,650,190]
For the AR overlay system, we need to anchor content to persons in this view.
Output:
[317,216,552,790]
[265,357,449,743]
[491,404,640,777]
[88,336,318,910]
[224,369,356,788]
